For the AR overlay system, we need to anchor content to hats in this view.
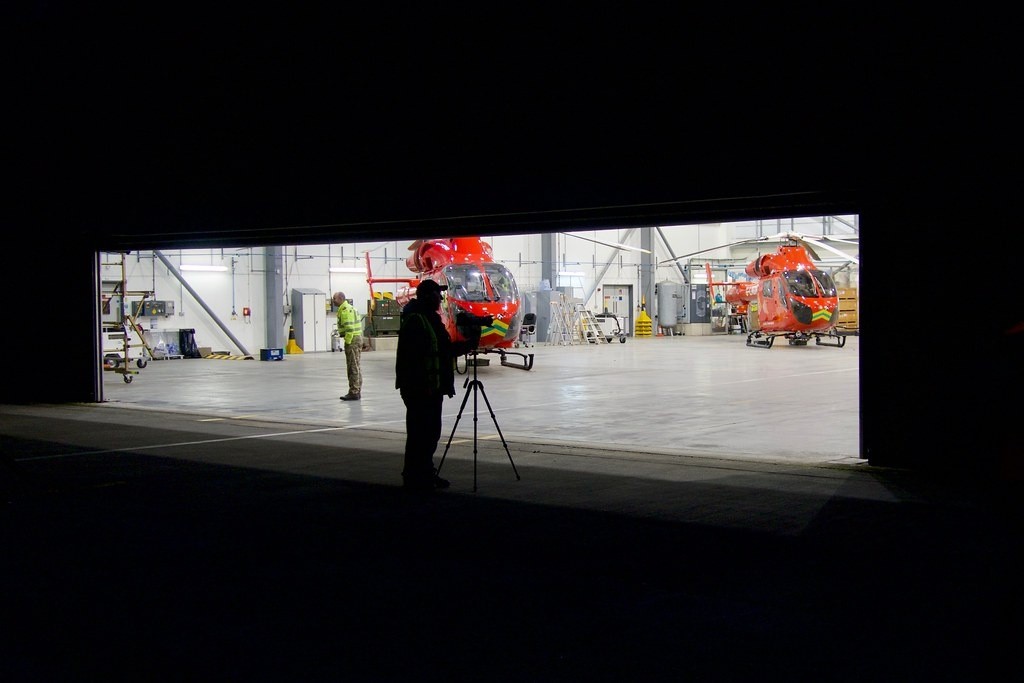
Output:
[416,279,448,296]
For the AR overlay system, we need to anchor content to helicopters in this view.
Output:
[365,233,651,374]
[656,228,859,351]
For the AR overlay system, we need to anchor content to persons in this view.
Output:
[333,290,364,401]
[395,278,481,491]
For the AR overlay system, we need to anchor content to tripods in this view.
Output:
[435,340,520,491]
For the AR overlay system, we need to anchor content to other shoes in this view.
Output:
[401,468,450,492]
[340,392,361,401]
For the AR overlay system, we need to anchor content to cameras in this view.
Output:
[456,313,496,328]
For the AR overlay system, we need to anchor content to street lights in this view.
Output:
[657,324,663,337]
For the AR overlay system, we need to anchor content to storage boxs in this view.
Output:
[260,348,284,361]
